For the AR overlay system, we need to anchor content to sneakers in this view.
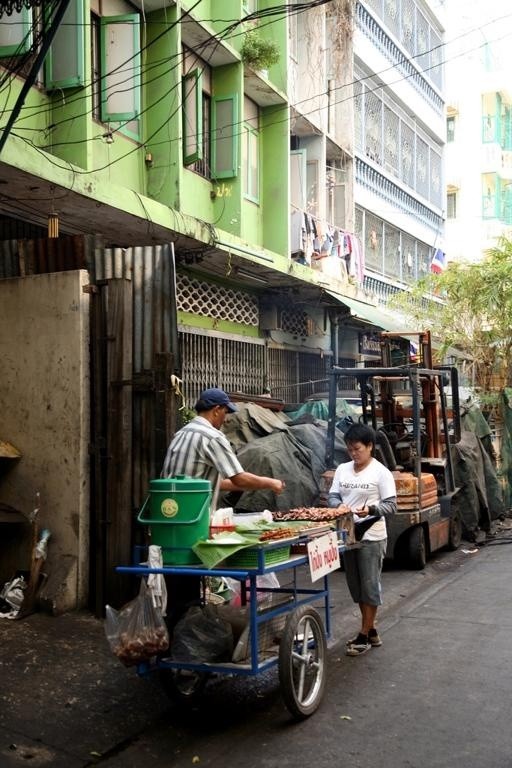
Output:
[347,629,382,646]
[346,633,371,656]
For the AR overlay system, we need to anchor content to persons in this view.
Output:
[326,424,398,655]
[156,388,286,519]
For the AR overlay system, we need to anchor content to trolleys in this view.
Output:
[116,526,349,717]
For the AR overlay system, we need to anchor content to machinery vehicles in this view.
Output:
[317,329,464,568]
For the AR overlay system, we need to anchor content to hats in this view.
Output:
[200,388,238,413]
